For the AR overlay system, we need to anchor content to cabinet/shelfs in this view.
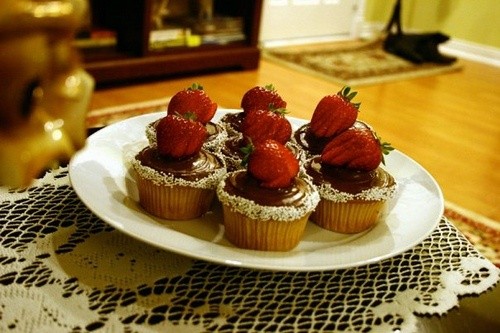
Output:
[56,0,266,88]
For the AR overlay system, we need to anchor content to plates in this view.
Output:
[67,107,445,271]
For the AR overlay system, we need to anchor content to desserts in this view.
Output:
[130,82,397,252]
